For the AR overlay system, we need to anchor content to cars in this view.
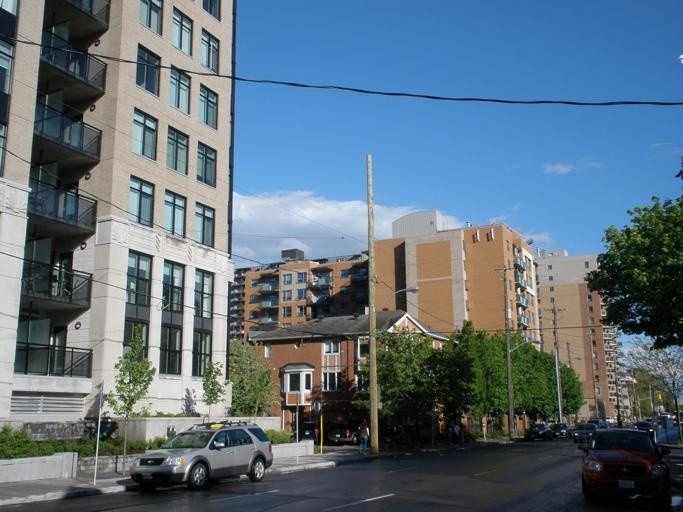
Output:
[521,407,682,512]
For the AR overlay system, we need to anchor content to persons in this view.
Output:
[356,418,370,457]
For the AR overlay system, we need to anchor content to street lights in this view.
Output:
[371,286,420,455]
[495,264,543,441]
[553,354,581,422]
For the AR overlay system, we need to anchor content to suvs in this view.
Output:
[315,402,371,446]
[127,418,273,492]
